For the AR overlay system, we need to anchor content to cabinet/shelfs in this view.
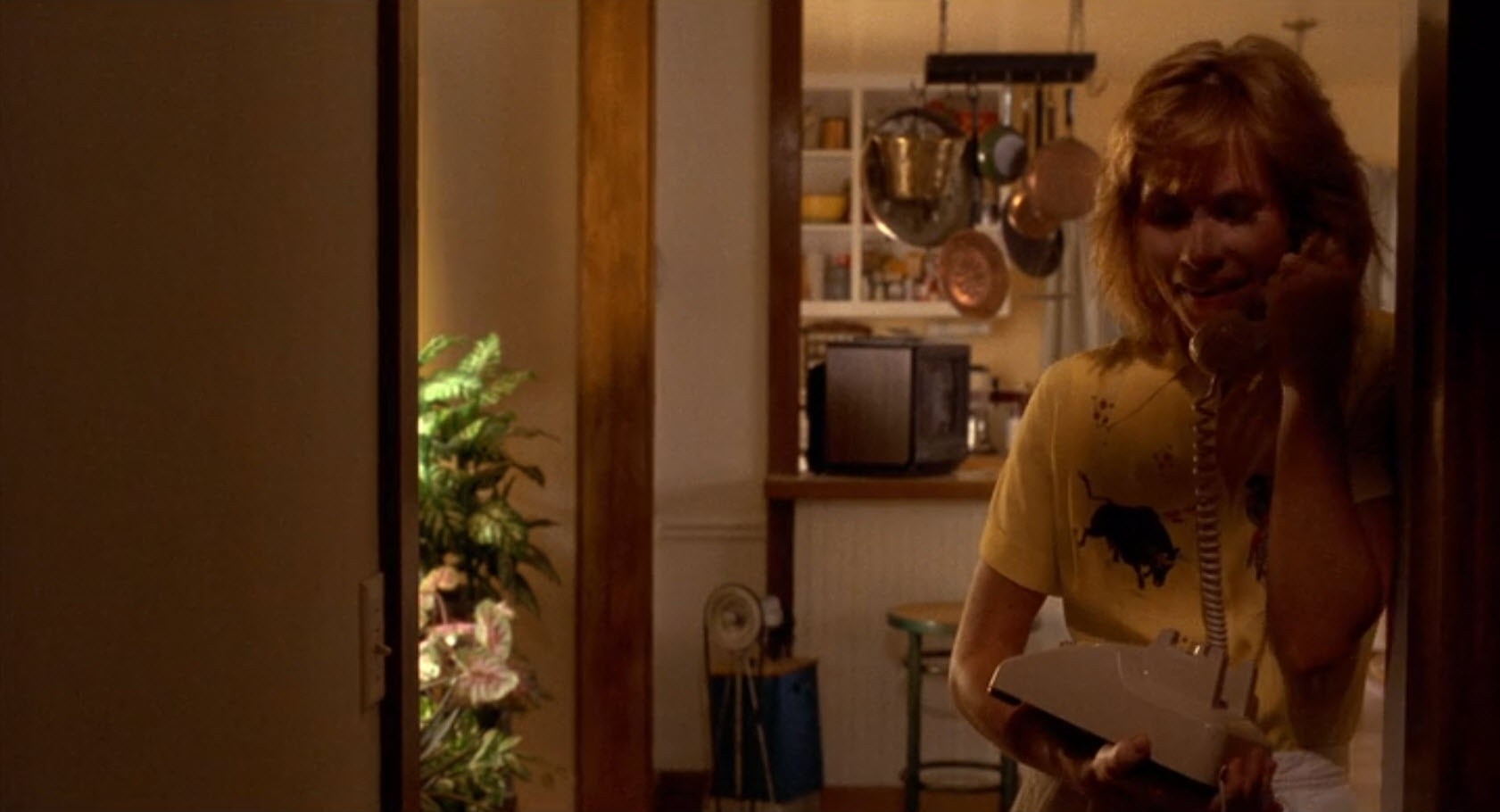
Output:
[801,66,1015,320]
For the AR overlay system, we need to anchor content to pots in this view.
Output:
[859,70,1103,319]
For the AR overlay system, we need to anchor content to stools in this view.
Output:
[888,598,1043,812]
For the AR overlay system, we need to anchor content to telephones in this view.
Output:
[1187,231,1339,372]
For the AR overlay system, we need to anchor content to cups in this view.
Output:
[799,193,848,225]
[800,113,849,150]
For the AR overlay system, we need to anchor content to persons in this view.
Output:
[948,32,1396,811]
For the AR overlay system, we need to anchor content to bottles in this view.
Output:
[963,363,1023,459]
[802,244,850,298]
